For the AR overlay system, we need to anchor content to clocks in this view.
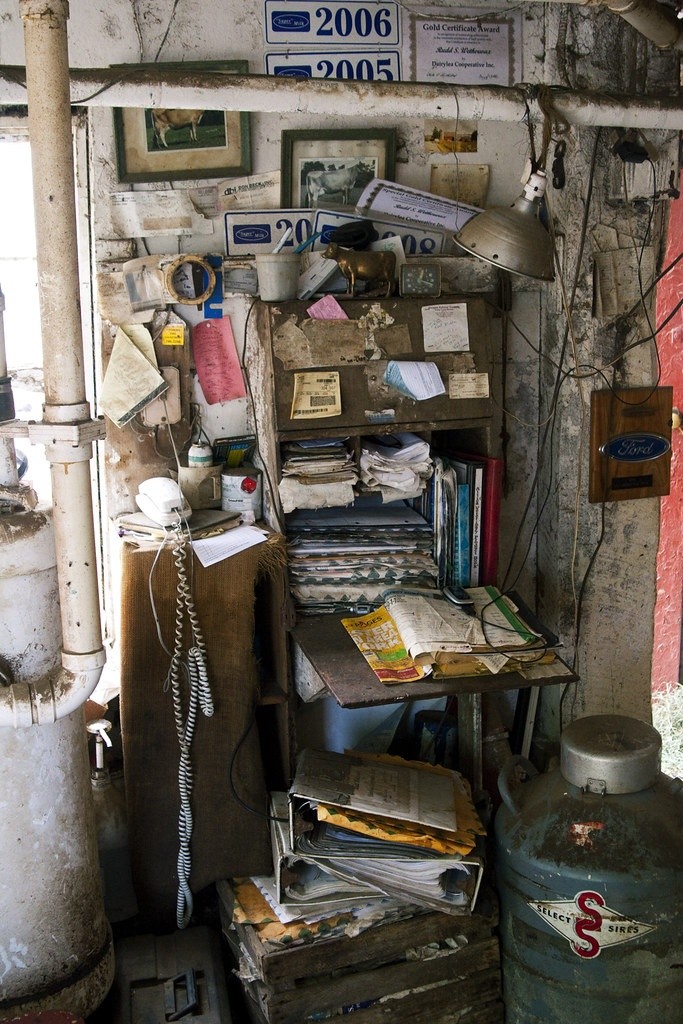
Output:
[399,262,443,298]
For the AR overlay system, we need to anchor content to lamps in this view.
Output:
[449,169,557,283]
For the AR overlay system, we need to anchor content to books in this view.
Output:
[280,453,566,683]
[115,512,243,542]
[270,748,485,919]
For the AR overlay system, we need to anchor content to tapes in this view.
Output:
[166,255,216,305]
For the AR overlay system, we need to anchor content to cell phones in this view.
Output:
[442,585,474,609]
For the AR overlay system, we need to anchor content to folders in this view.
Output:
[288,748,457,860]
[267,791,392,907]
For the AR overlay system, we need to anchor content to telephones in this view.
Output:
[135,477,193,528]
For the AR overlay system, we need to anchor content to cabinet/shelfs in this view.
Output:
[122,293,579,927]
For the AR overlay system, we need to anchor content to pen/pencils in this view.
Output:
[320,858,486,916]
[293,231,322,255]
[271,228,293,254]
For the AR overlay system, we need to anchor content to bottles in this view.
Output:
[189,442,213,467]
[491,715,683,1024]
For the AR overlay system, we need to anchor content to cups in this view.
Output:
[254,252,300,302]
[177,461,223,509]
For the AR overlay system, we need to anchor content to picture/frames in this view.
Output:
[108,60,252,185]
[280,128,397,209]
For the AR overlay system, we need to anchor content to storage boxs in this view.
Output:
[211,880,505,1024]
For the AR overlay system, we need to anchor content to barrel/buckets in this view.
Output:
[220,467,262,521]
